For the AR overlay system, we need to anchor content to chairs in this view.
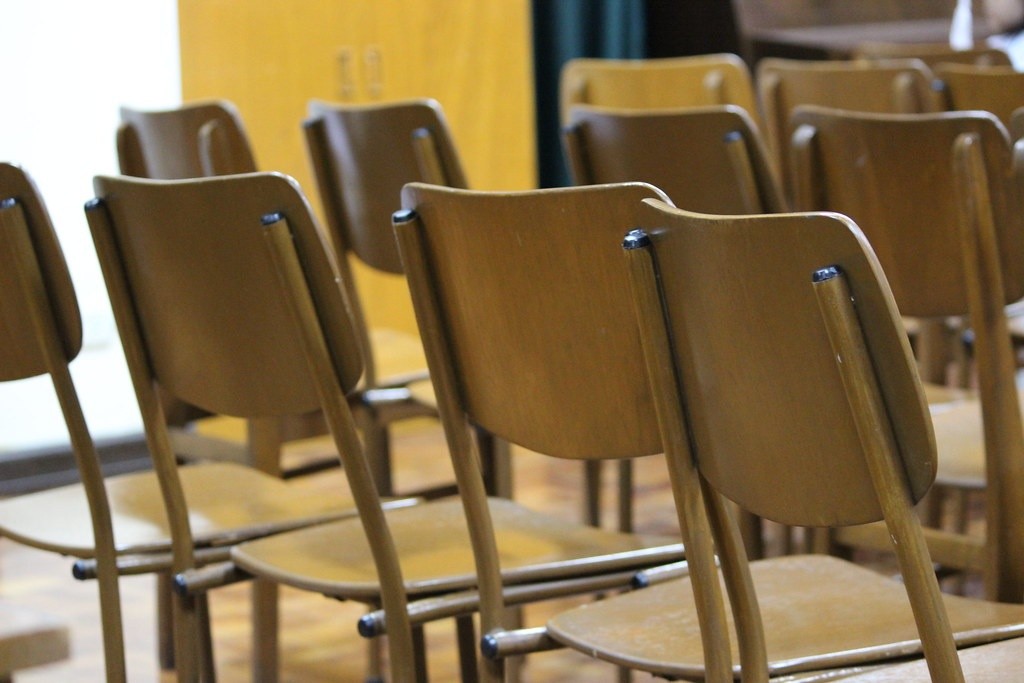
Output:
[0,38,1024,683]
